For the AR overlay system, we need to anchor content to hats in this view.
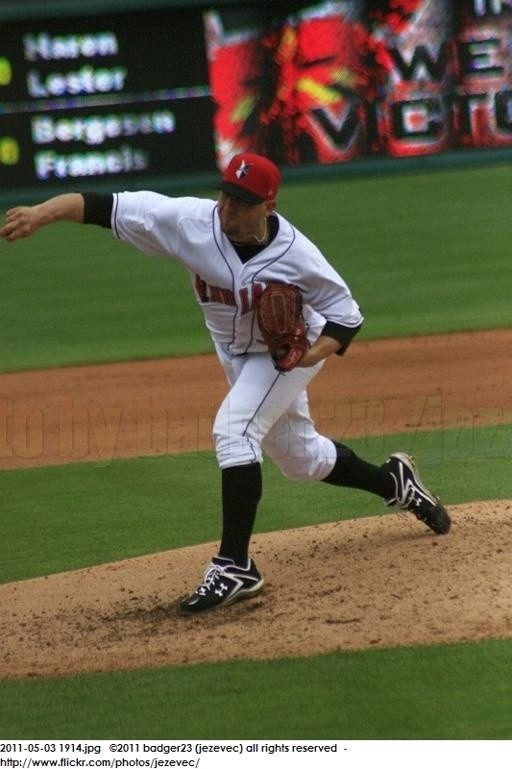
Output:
[215,155,282,205]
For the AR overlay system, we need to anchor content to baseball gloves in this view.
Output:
[257,283,311,376]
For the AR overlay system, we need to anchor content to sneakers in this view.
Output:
[380,454,450,533]
[178,556,264,617]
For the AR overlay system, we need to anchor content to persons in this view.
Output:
[1,151,449,610]
